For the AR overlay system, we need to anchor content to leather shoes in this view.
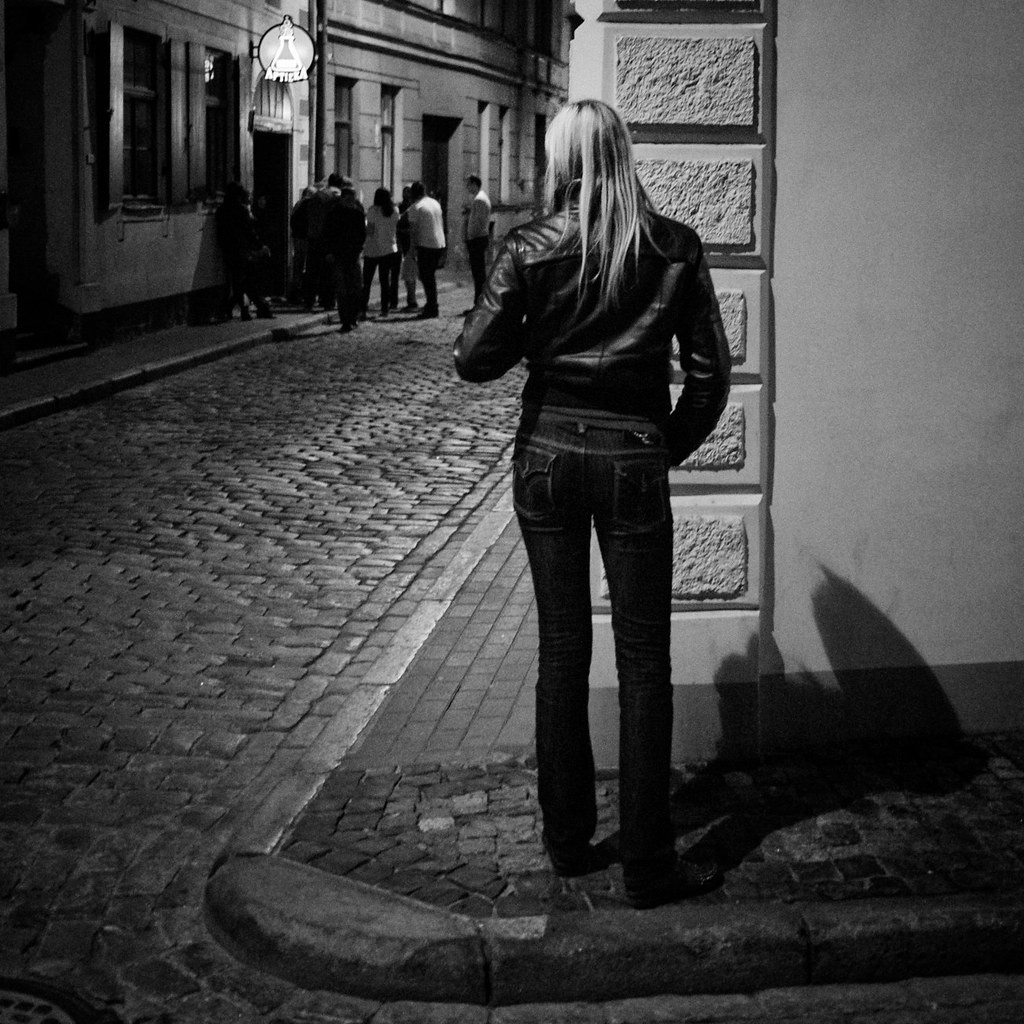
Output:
[545,846,589,874]
[625,862,719,910]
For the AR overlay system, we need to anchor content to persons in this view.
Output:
[396,184,418,309]
[462,173,491,313]
[452,100,732,908]
[408,182,445,318]
[289,173,367,330]
[362,187,400,317]
[217,182,275,319]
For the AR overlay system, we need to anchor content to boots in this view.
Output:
[240,305,252,321]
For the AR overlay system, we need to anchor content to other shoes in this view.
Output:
[417,305,437,319]
[389,305,396,309]
[359,315,366,321]
[335,327,352,333]
[401,305,418,312]
[380,312,387,317]
